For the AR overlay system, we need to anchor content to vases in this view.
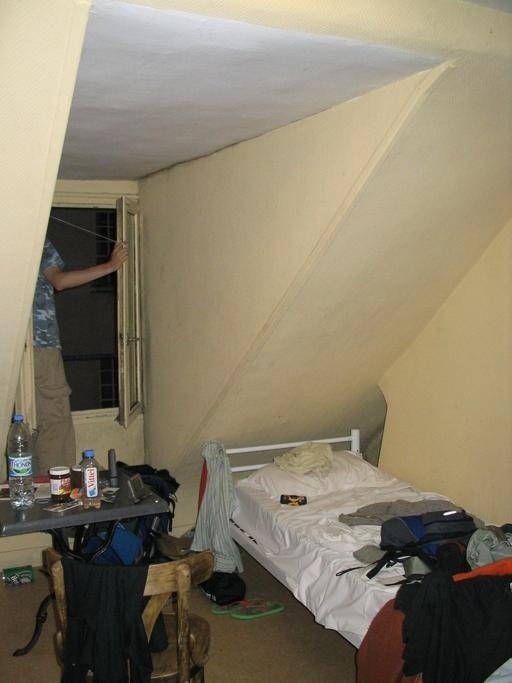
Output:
[211,427,511,682]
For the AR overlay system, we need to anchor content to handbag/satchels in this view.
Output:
[379,510,478,569]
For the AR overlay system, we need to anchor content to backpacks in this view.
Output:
[79,462,180,570]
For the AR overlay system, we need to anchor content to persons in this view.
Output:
[31,237,130,475]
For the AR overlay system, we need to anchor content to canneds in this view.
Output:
[48,467,72,503]
[3,565,33,586]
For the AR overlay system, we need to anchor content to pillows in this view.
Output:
[241,449,396,502]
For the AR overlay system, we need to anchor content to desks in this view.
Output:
[1,470,170,657]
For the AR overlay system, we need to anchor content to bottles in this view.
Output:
[80,448,101,502]
[7,415,34,510]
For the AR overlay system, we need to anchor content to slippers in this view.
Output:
[210,599,287,621]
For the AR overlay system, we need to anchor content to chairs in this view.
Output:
[45,543,215,683]
